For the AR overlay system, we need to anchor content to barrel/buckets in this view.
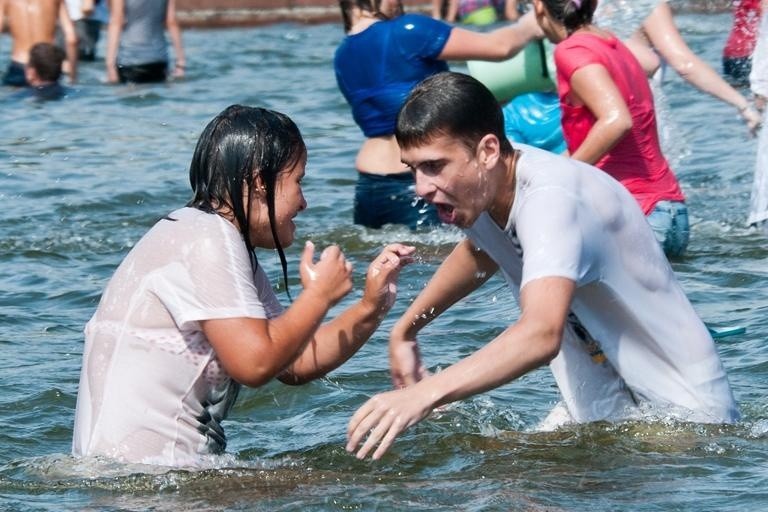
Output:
[463,38,554,105]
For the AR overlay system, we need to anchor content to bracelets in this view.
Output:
[176,62,187,71]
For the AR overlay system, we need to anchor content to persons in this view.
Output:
[375,0,403,19]
[743,34,768,230]
[65,0,109,61]
[23,41,73,100]
[533,1,692,261]
[429,0,520,27]
[0,0,81,88]
[105,0,187,89]
[591,1,760,180]
[343,70,739,468]
[719,1,765,86]
[72,101,418,467]
[331,0,543,237]
